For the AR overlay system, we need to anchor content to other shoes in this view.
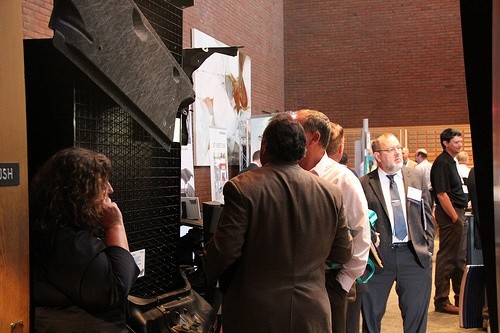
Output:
[435,303,459,314]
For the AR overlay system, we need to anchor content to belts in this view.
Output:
[391,243,409,247]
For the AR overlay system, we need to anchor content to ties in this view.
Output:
[386,173,407,241]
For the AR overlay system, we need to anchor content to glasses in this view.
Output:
[377,146,403,153]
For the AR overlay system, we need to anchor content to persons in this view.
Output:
[402,145,418,168]
[208,106,373,333]
[413,147,433,210]
[359,131,436,333]
[31,146,142,333]
[430,127,482,314]
